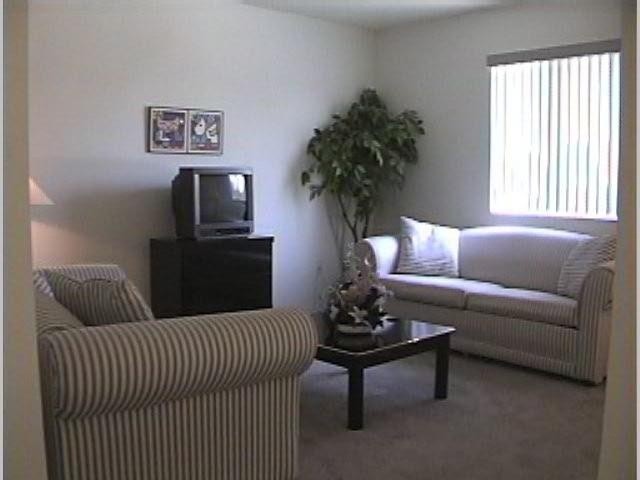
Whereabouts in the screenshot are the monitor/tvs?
[171,166,255,239]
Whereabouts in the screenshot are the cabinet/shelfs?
[148,234,275,317]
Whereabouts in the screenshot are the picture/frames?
[187,109,225,155]
[147,106,188,155]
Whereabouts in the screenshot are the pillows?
[394,215,460,277]
[558,232,616,297]
[42,268,155,327]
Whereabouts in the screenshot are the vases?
[332,337,385,353]
[332,323,396,336]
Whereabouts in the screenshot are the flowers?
[324,242,396,330]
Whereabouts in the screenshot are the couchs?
[361,223,618,385]
[34,262,319,480]
